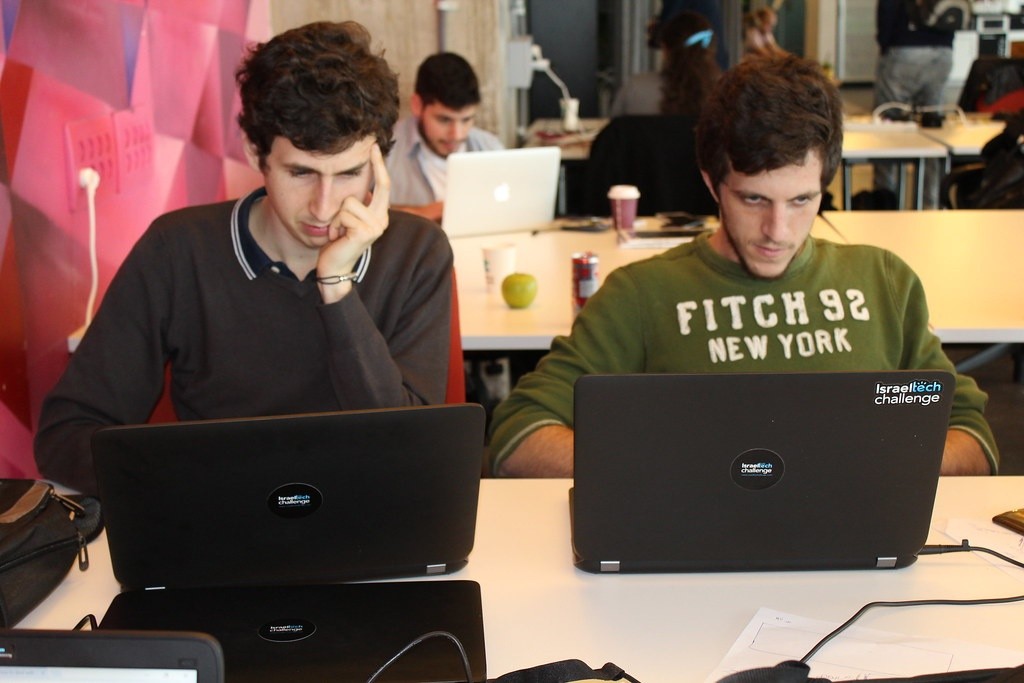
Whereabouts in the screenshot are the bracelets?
[315,273,357,284]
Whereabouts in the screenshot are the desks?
[522,110,948,216]
[451,214,851,415]
[821,207,1024,378]
[899,107,1024,210]
[6,474,1024,683]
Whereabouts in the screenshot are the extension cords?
[843,119,919,134]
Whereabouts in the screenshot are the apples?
[502,272,537,308]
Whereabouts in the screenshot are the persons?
[34,21,454,495]
[385,52,505,399]
[743,8,790,58]
[568,12,722,217]
[872,0,964,210]
[485,55,1000,478]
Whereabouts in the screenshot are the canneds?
[572,251,600,310]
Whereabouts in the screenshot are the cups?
[607,185,641,230]
[560,99,580,131]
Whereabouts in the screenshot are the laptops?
[0,405,489,683]
[440,146,561,237]
[568,370,955,574]
